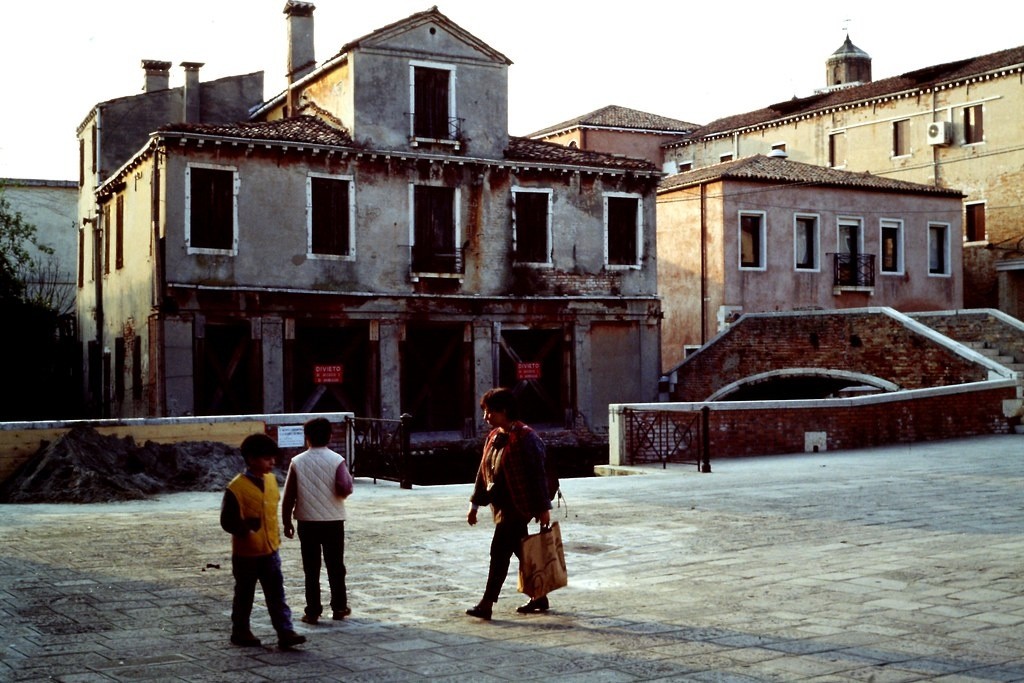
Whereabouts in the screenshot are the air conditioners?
[928,122,952,147]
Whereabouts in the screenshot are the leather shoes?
[465,605,492,620]
[516,596,549,613]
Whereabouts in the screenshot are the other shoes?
[301,615,317,625]
[334,606,351,620]
[230,630,260,647]
[278,631,306,649]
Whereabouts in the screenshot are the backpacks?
[485,427,560,502]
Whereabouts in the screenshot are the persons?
[465,388,553,620]
[282,417,354,624]
[220,434,306,649]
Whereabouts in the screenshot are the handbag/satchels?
[517,522,567,600]
